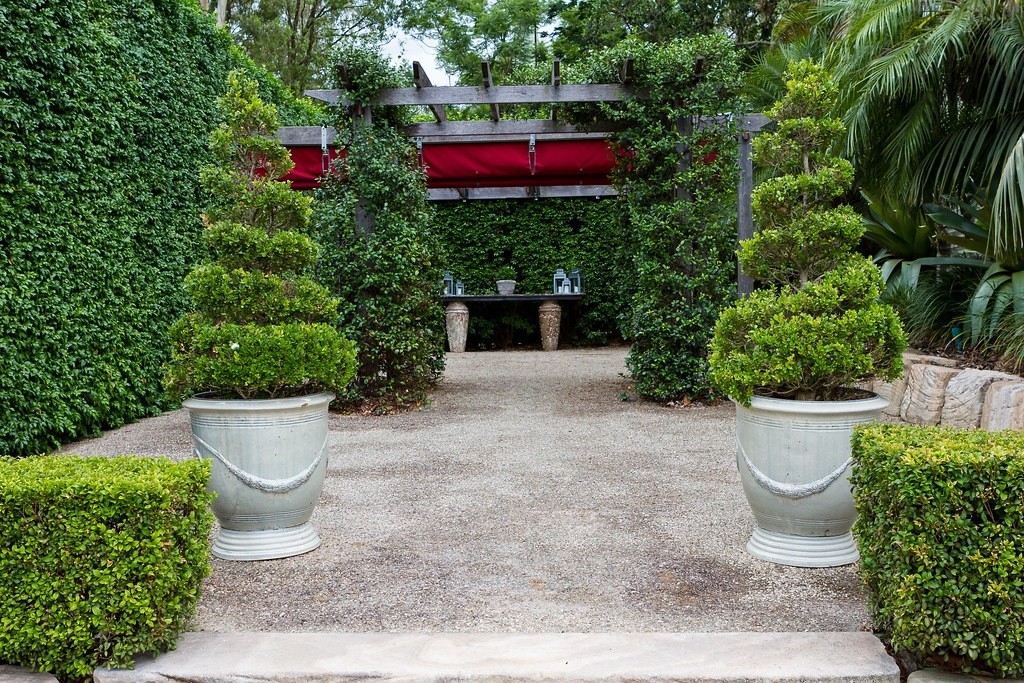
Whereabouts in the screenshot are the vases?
[538,301,562,352]
[445,301,469,353]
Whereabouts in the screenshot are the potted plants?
[169,66,358,562]
[493,266,517,296]
[707,57,909,569]
